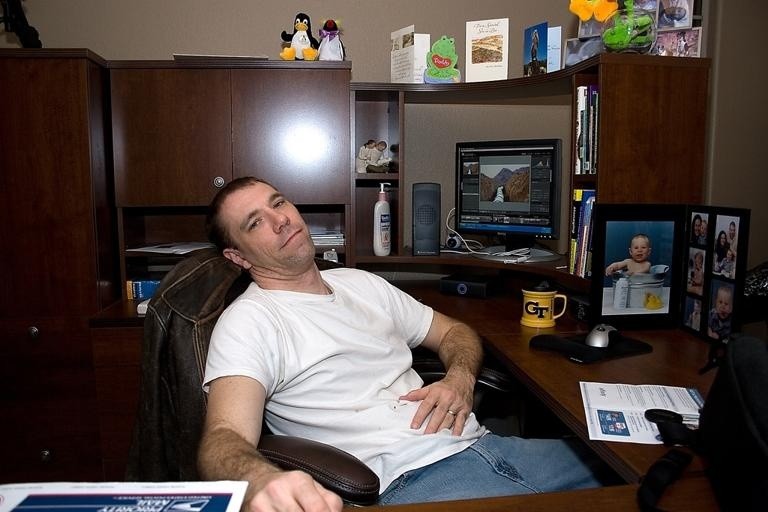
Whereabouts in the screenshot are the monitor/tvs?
[454,138,562,264]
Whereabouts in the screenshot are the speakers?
[412,182,441,256]
[440,273,494,297]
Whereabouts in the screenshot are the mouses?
[584,323,618,348]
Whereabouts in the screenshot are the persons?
[675,30,689,58]
[193,177,609,512]
[659,5,688,28]
[355,139,377,174]
[605,233,652,278]
[682,209,743,346]
[530,30,541,76]
[365,140,388,166]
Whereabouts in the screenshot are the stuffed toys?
[315,18,347,63]
[278,13,319,61]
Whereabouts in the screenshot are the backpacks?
[634,266,768,512]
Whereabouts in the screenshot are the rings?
[446,410,457,417]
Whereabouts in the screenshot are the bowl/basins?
[610,273,666,309]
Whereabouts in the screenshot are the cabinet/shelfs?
[85,308,145,482]
[101,53,354,301]
[355,53,712,295]
[0,48,120,483]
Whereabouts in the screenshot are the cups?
[519,287,567,330]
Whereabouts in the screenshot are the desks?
[334,271,732,510]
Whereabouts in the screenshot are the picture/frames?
[592,202,752,347]
[562,0,703,70]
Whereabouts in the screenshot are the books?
[574,86,600,175]
[307,229,345,247]
[567,188,596,279]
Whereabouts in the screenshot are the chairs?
[137,245,513,506]
